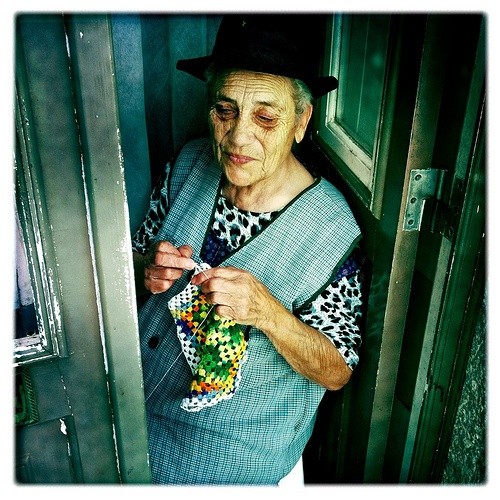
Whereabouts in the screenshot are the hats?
[174,15,342,101]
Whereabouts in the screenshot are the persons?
[130,15,366,485]
